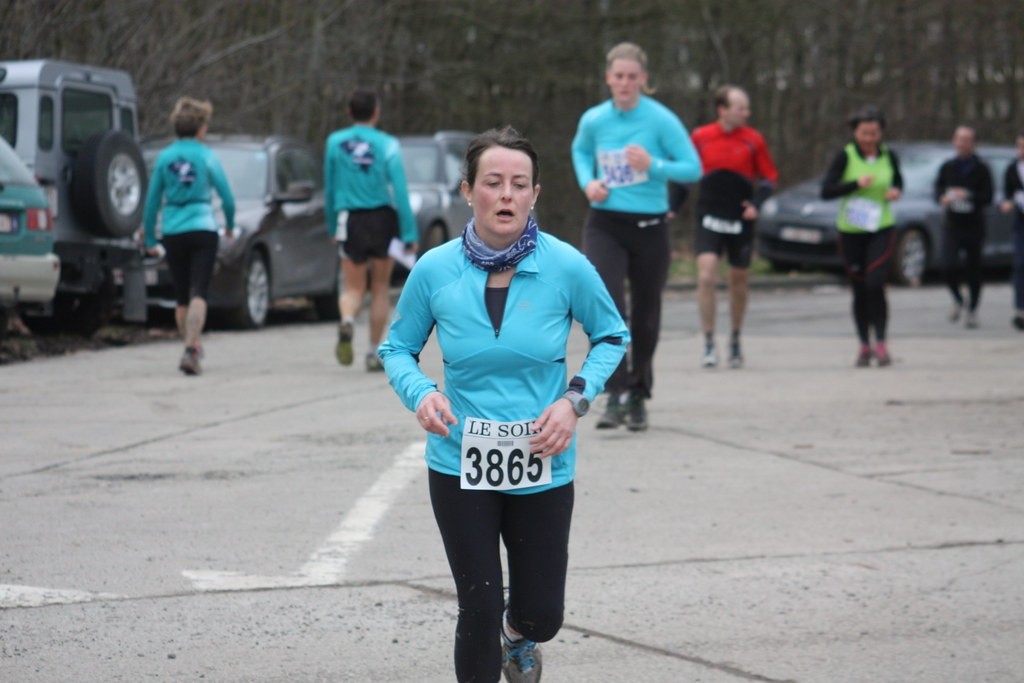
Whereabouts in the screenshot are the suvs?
[0,58,150,337]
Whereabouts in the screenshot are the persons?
[378,125,632,683]
[669,84,781,368]
[142,99,235,376]
[323,91,420,372]
[820,110,906,367]
[1001,133,1024,330]
[935,125,995,330]
[571,42,702,432]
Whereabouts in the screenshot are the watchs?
[563,391,590,417]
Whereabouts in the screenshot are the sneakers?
[627,395,649,431]
[499,587,542,683]
[181,343,204,375]
[597,396,624,428]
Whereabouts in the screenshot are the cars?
[366,128,480,290]
[753,140,1021,288]
[107,133,342,330]
[0,132,61,310]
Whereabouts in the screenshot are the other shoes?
[875,342,889,368]
[703,345,721,366]
[965,309,978,327]
[366,350,381,372]
[948,299,964,320]
[1014,317,1024,328]
[336,321,354,365]
[857,344,872,367]
[730,349,744,370]
